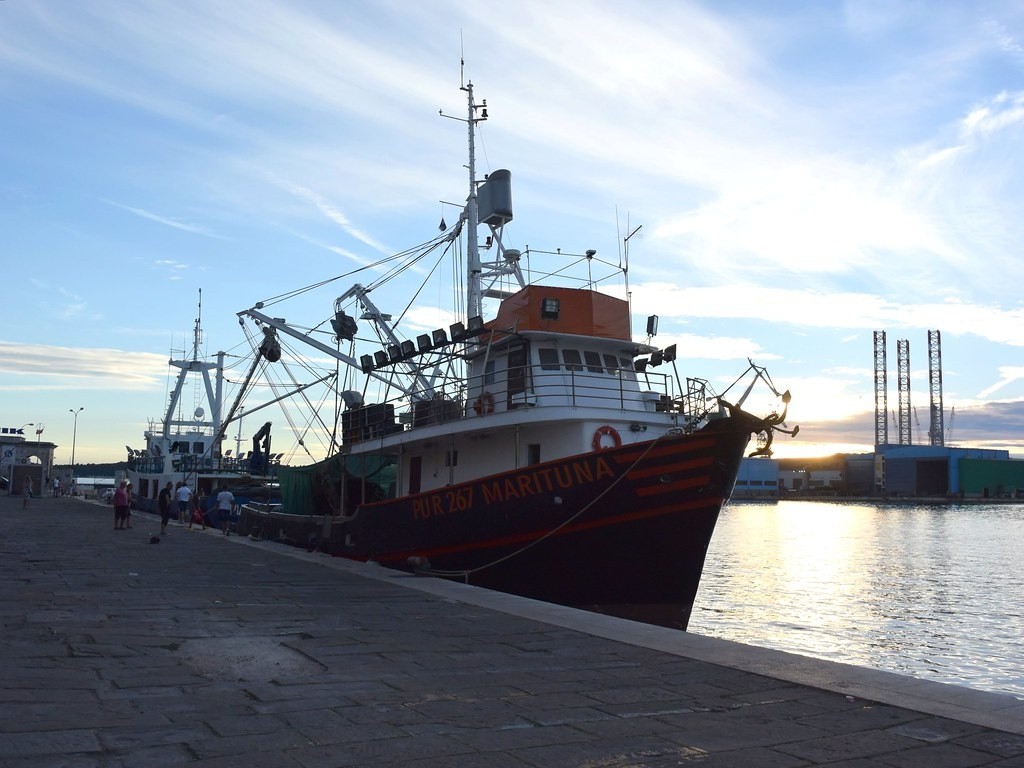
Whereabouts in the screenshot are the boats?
[239,57,799,630]
[114,288,282,531]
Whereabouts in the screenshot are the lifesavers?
[476,391,495,416]
[592,426,622,452]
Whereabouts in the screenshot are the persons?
[69,479,76,499]
[156,482,174,536]
[215,484,236,537]
[53,476,60,498]
[121,483,137,529]
[23,476,33,511]
[57,479,63,496]
[112,480,129,530]
[176,482,192,524]
